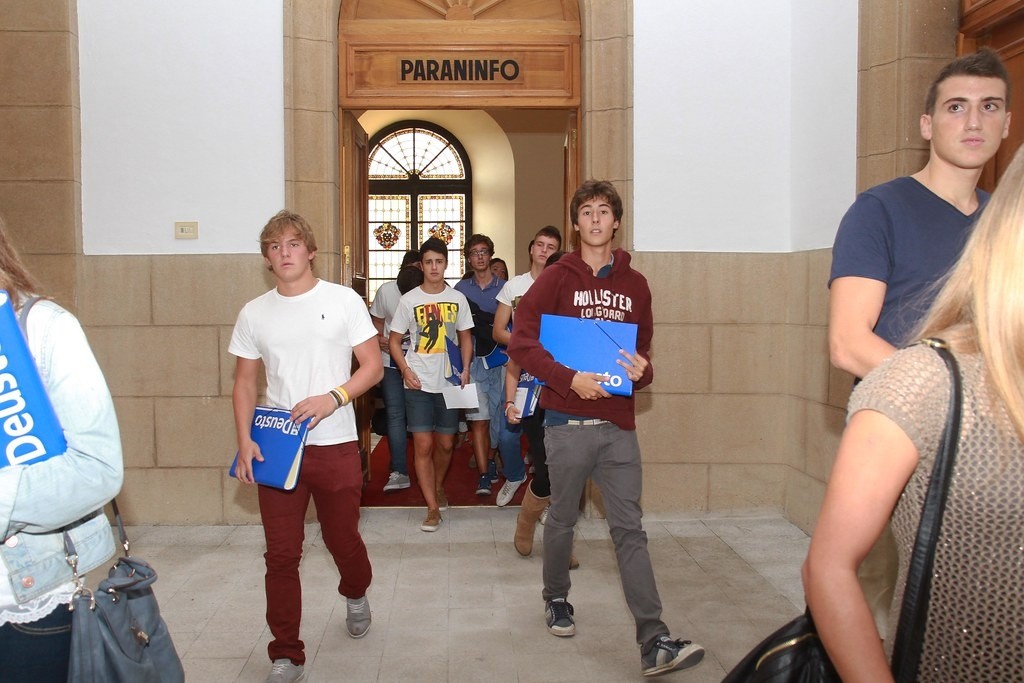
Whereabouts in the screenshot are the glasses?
[467,247,491,256]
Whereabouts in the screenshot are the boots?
[570,555,581,571]
[514,478,552,557]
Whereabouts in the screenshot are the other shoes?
[458,414,468,433]
[529,464,536,473]
[524,453,534,464]
[467,453,478,469]
[540,505,548,525]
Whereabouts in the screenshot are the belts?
[567,419,613,427]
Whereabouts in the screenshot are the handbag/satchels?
[723,614,843,683]
[57,557,186,683]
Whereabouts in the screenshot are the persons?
[0,217,124,683]
[828,49,1011,387]
[802,142,1024,683]
[228,209,384,683]
[507,180,704,676]
[369,226,579,570]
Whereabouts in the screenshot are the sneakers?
[383,470,412,495]
[476,474,493,497]
[262,659,306,683]
[420,508,442,532]
[640,633,707,678]
[344,596,374,639]
[543,594,576,637]
[488,458,500,484]
[435,486,449,511]
[495,474,527,507]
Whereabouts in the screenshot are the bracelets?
[328,386,349,408]
[505,404,514,416]
[401,366,411,377]
[506,401,514,404]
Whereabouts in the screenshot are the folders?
[482,322,513,370]
[514,369,541,419]
[0,290,68,467]
[539,313,639,396]
[230,406,312,490]
[444,335,463,386]
